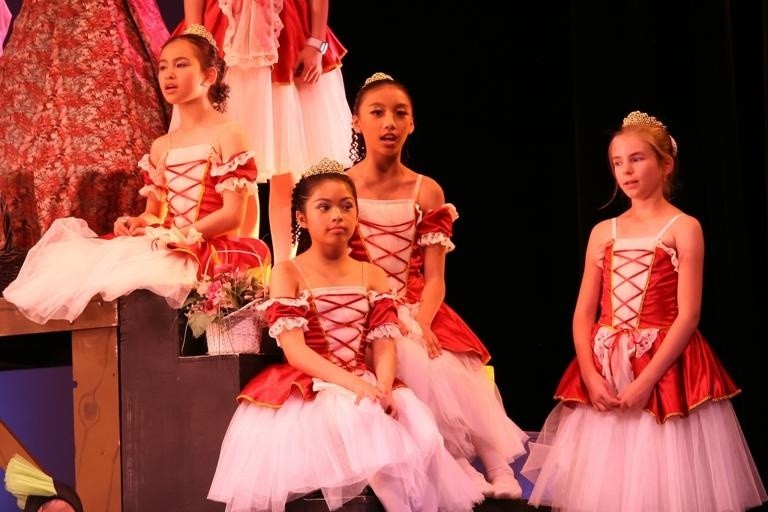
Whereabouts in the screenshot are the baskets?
[200,250,271,354]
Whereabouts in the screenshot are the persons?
[521,111,765,512]
[341,72,530,499]
[173,1,356,263]
[1,0,179,257]
[4,24,256,324]
[206,157,493,512]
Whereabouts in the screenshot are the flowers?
[181,262,270,357]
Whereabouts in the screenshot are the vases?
[205,298,263,354]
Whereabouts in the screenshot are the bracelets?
[305,37,329,56]
